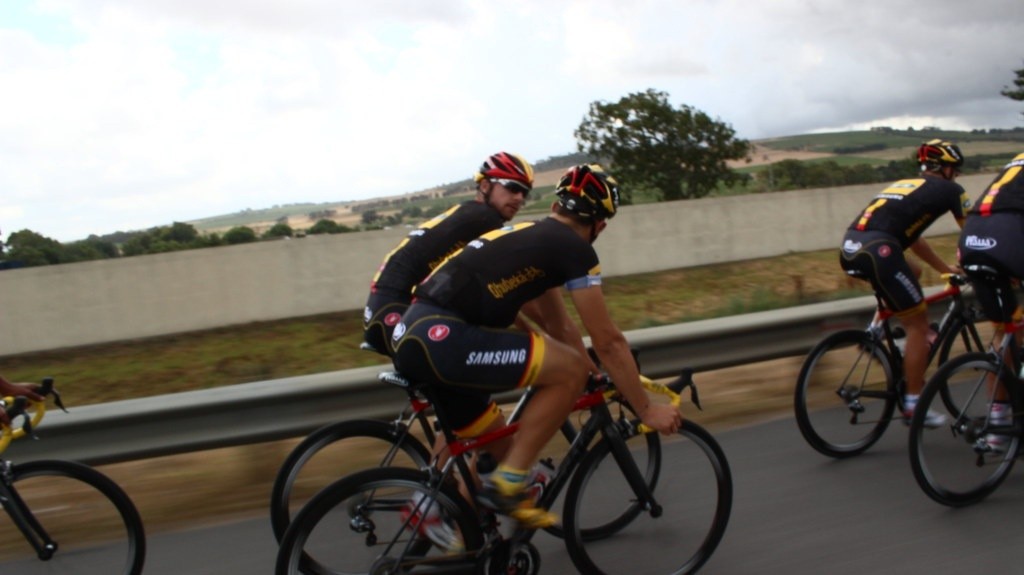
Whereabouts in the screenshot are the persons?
[956,152,1024,456]
[0,375,46,432]
[391,162,686,557]
[840,139,974,428]
[363,150,602,557]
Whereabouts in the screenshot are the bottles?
[476,452,498,487]
[927,323,939,350]
[892,327,906,357]
[533,457,555,498]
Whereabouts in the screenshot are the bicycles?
[793,273,1004,459]
[270,340,662,575]
[909,322,1023,509]
[274,366,734,575]
[0,375,147,575]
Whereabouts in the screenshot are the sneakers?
[400,490,456,552]
[444,529,465,558]
[987,419,1014,454]
[858,327,891,365]
[472,478,561,530]
[902,405,946,428]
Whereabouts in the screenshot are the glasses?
[489,178,531,199]
[947,165,962,177]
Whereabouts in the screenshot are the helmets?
[474,151,535,190]
[553,162,621,220]
[916,138,965,167]
